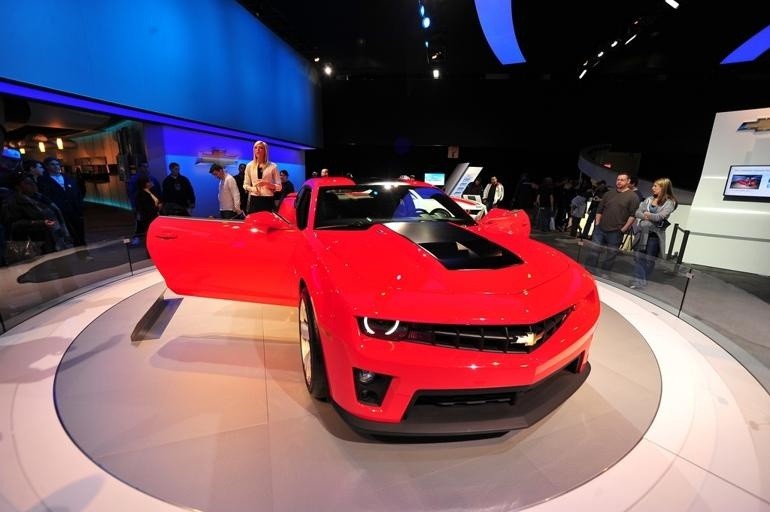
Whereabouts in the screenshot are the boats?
[194,145,243,168]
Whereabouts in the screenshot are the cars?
[359,176,488,221]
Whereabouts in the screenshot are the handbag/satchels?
[549,217,556,231]
[0,240,37,267]
[619,233,635,252]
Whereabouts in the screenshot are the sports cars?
[141,169,606,445]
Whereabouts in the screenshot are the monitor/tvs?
[721,165,770,202]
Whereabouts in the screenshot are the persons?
[320,168,329,177]
[1,138,296,266]
[468,168,679,288]
